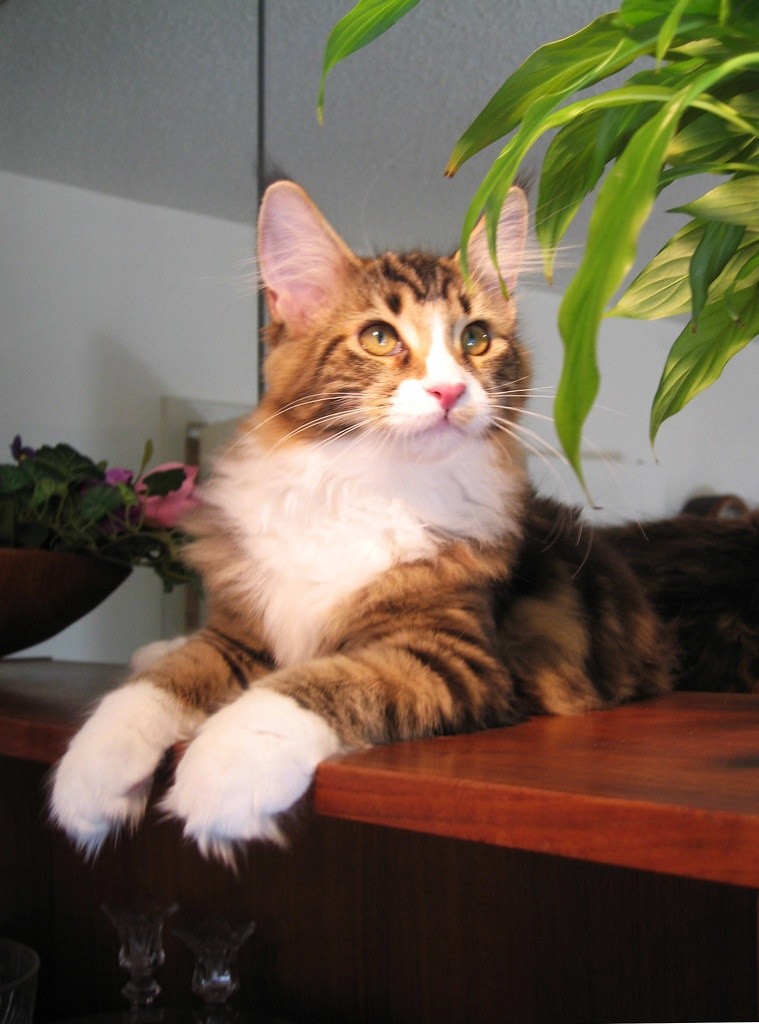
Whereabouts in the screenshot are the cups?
[100,901,181,1024]
[177,909,257,1024]
[0,938,41,1024]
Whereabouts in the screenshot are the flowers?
[0,434,206,600]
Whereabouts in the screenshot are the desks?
[0,660,759,1022]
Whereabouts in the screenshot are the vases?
[0,548,133,660]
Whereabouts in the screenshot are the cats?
[36,160,759,881]
